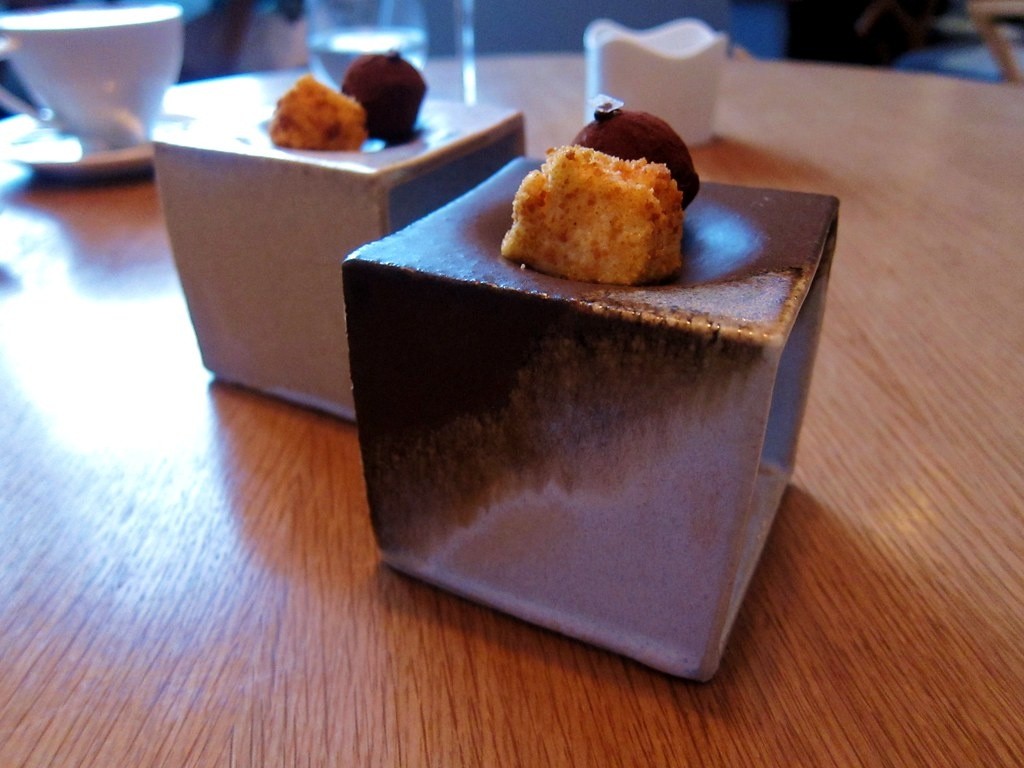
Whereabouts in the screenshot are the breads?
[499,144,685,286]
[267,73,367,150]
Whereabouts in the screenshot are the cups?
[585,19,728,145]
[0,7,184,145]
[375,0,478,109]
[305,0,430,94]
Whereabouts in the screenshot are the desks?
[0,53,1024,768]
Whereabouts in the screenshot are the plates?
[5,136,154,180]
